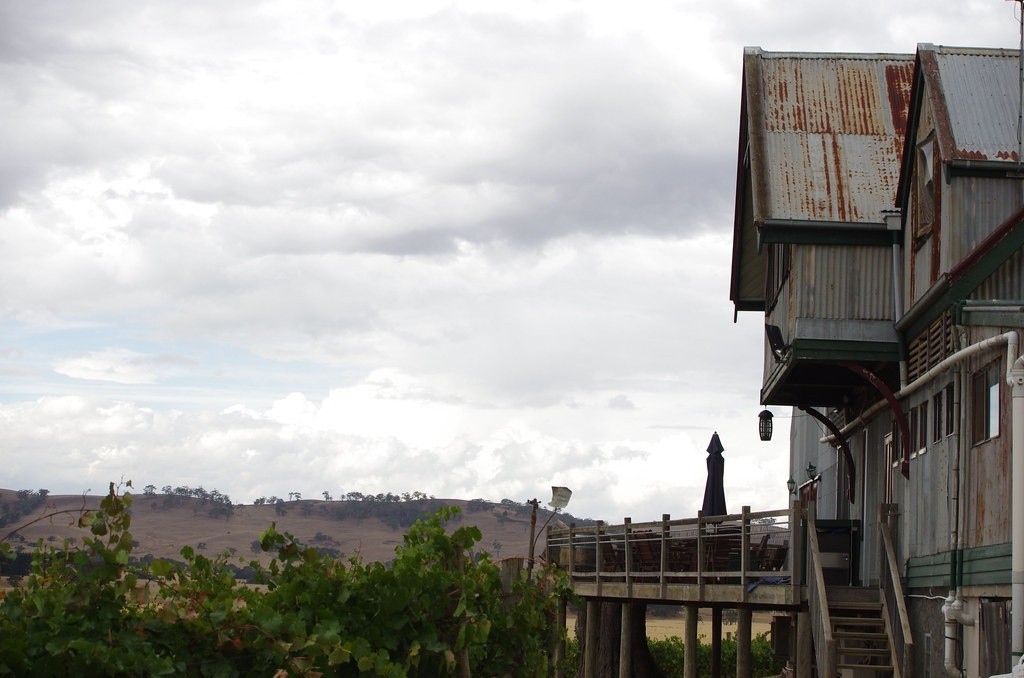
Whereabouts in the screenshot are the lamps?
[805,461,822,482]
[787,475,798,495]
[758,405,774,441]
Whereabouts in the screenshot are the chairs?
[601,523,788,584]
